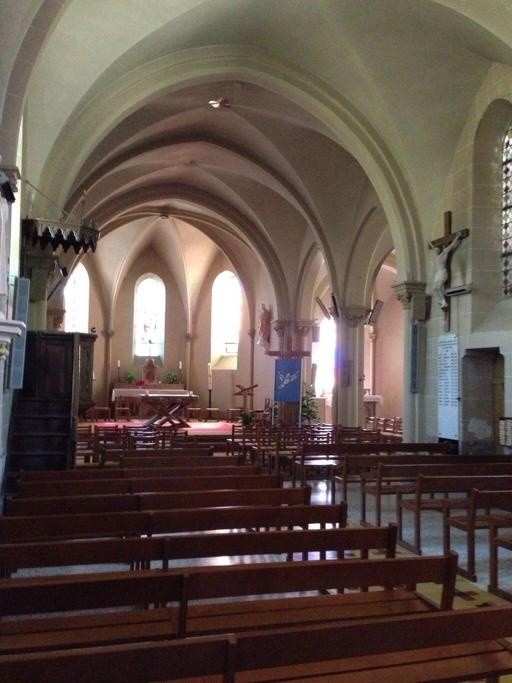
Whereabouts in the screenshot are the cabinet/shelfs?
[26,330,99,413]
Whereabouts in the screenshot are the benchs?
[1,383,512,683]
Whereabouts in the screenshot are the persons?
[258,301,270,342]
[426,231,461,308]
